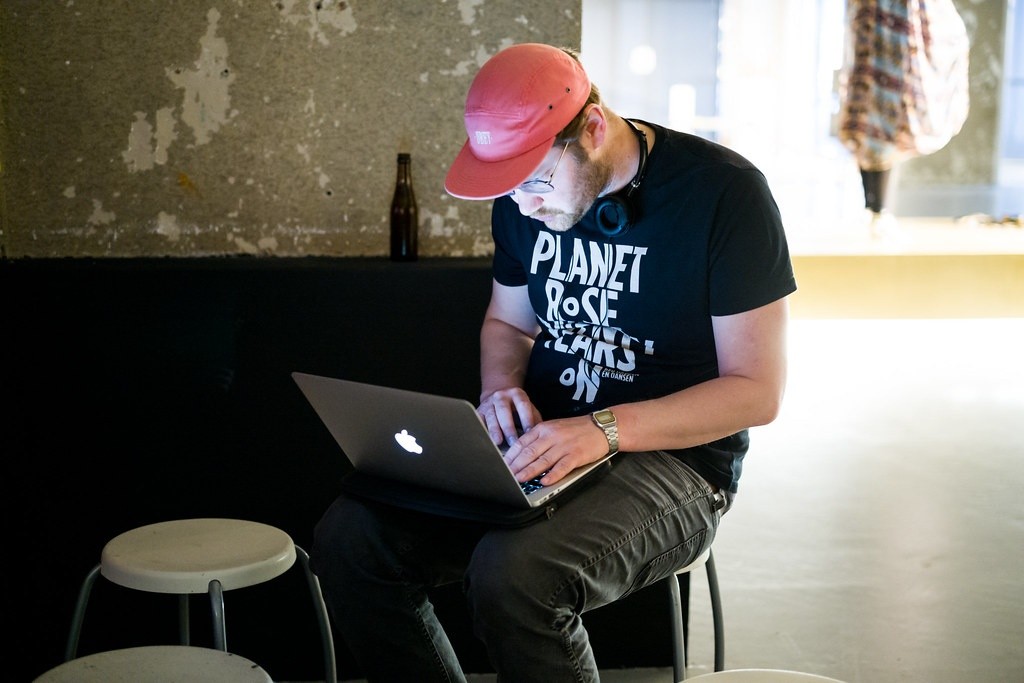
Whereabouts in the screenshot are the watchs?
[589,409,619,455]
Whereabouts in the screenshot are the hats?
[444,43,591,201]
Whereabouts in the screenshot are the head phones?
[596,116,649,236]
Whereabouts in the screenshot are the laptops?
[291,372,620,507]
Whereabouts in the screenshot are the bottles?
[389,153,418,259]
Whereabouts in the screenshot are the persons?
[311,42,797,683]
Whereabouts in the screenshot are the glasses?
[505,141,570,195]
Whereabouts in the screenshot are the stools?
[65,517,338,683]
[667,544,725,683]
[677,668,848,683]
[31,645,275,683]
